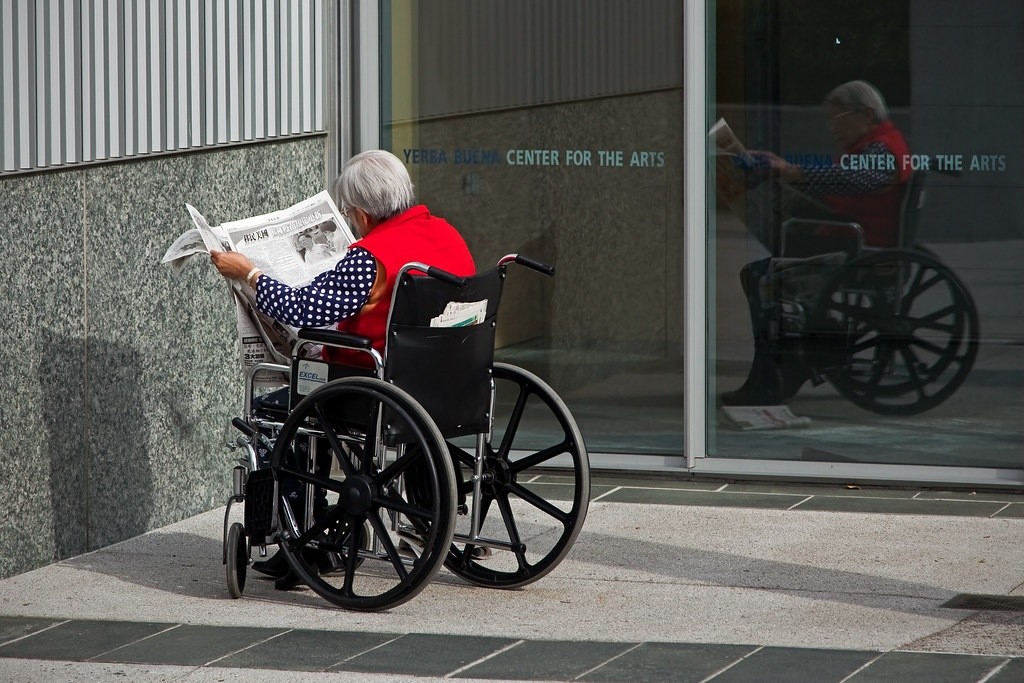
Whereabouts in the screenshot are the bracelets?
[247,268,262,285]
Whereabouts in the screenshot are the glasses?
[823,107,858,131]
[340,206,356,217]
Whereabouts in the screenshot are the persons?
[210,150,477,582]
[298,220,349,263]
[721,82,913,406]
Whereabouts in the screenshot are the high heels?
[745,358,828,406]
[253,542,339,590]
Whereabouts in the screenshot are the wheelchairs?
[221,253,590,615]
[758,159,979,418]
